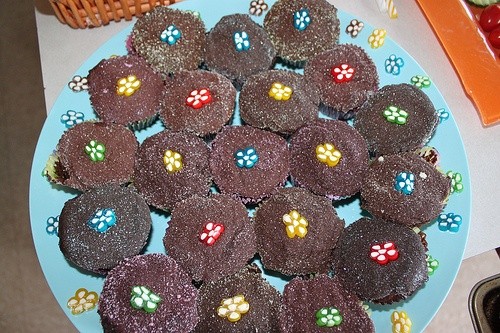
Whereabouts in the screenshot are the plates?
[27,0,476,333]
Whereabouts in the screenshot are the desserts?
[57,0,449,333]
